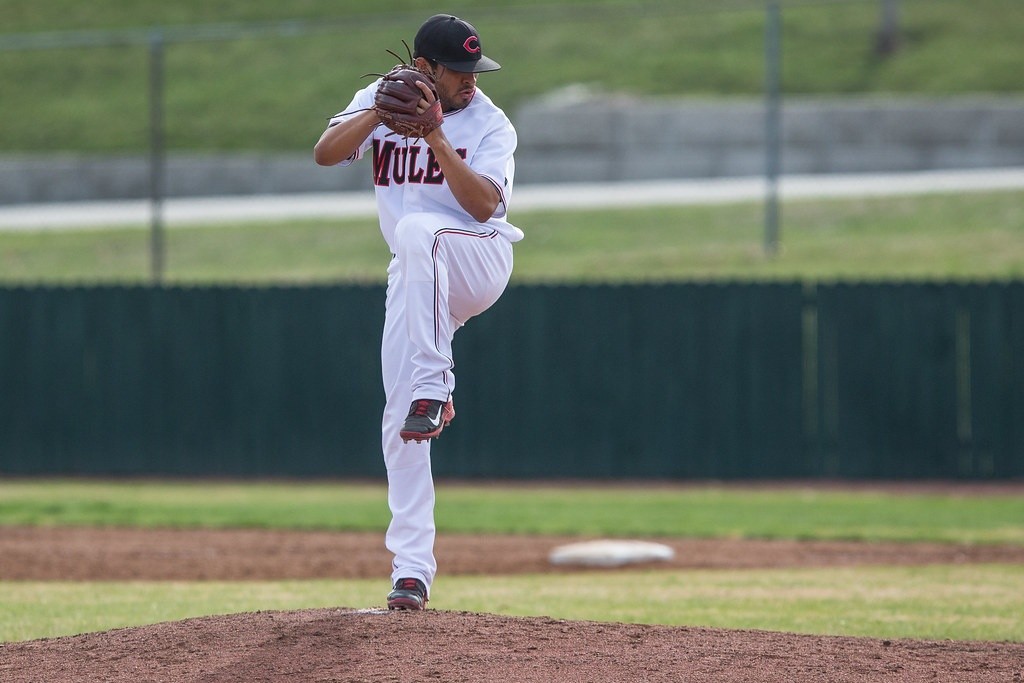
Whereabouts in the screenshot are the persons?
[314,14,524,612]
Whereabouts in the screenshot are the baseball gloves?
[374,63,444,139]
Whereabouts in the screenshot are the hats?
[413,14,502,73]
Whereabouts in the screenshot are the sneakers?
[400,399,455,444]
[387,578,429,610]
